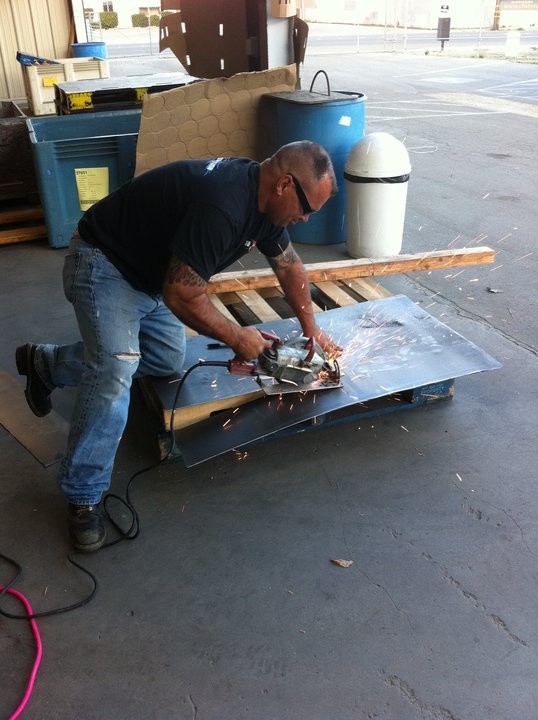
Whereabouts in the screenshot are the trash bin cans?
[343,131,413,259]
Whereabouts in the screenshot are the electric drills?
[226,329,332,389]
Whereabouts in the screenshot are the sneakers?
[64,496,107,553]
[15,341,55,418]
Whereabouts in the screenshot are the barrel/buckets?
[70,42,106,59]
[258,90,366,245]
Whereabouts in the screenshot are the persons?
[12,141,338,552]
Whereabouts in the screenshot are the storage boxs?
[22,56,110,116]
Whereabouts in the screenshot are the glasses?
[288,173,317,218]
